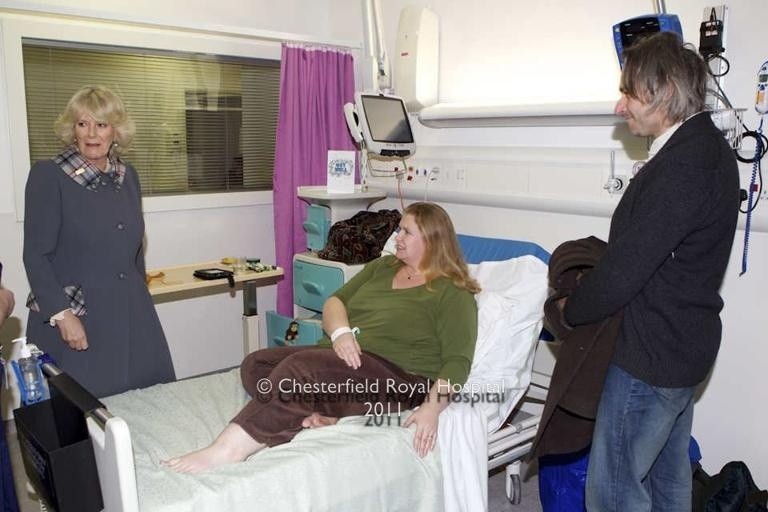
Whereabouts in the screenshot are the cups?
[231,257,247,274]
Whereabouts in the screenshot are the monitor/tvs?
[613,14,683,72]
[354,92,416,160]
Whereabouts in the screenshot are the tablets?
[195,268,233,278]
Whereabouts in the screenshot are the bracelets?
[329,326,356,343]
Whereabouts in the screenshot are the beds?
[35,227,579,512]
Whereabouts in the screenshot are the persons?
[158,202,483,475]
[1,261,16,327]
[547,30,741,511]
[27,83,176,399]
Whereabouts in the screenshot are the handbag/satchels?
[319,209,402,264]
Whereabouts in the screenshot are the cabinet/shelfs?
[266,184,388,366]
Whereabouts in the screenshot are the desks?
[141,257,284,367]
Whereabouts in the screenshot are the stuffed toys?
[284,321,299,341]
